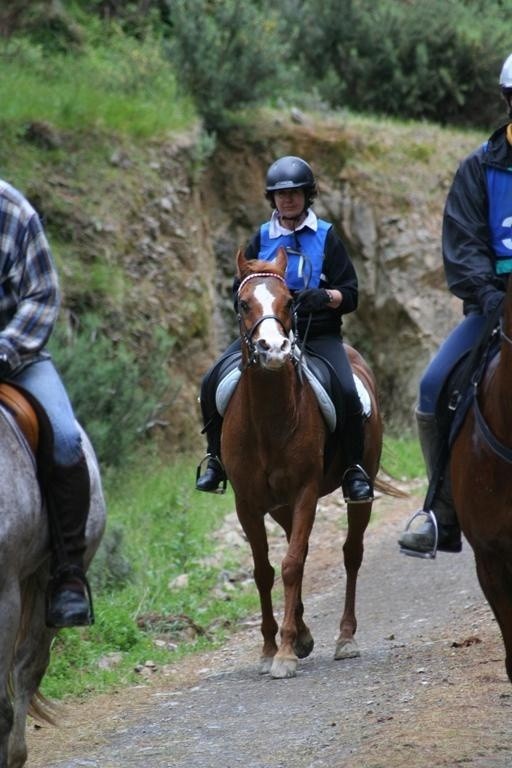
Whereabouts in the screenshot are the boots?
[45,496,93,628]
[339,406,375,506]
[398,408,462,553]
[196,425,220,491]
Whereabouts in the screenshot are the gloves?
[483,290,506,320]
[292,287,329,312]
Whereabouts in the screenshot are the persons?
[1,177,92,627]
[397,52,511,553]
[196,156,374,503]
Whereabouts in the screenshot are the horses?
[208,242,413,680]
[0,386,111,766]
[441,296,511,685]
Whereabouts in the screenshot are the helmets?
[266,156,315,189]
[499,52,512,88]
